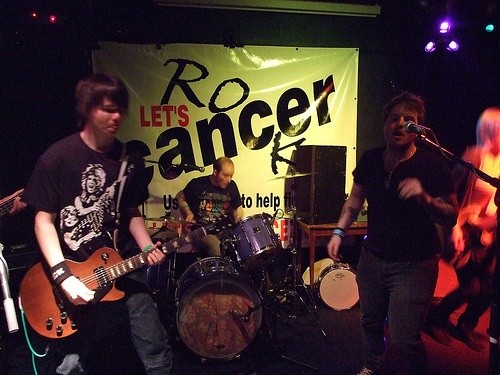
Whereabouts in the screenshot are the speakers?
[293,146,347,225]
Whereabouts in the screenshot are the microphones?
[184,164,206,172]
[269,211,278,226]
[231,308,249,323]
[404,121,431,133]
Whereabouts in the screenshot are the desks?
[294,220,369,290]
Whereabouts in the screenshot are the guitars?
[18,215,237,339]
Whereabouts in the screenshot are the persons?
[29,72,177,375]
[10,197,27,213]
[176,157,243,257]
[327,92,459,375]
[429,107,500,352]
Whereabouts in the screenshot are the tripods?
[246,177,330,375]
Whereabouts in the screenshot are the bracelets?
[332,228,346,239]
[50,261,73,285]
[182,206,189,209]
[144,245,153,252]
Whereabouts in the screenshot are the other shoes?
[421,320,453,345]
[356,366,376,375]
[454,316,485,352]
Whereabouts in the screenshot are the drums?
[220,214,282,273]
[301,257,360,312]
[176,258,263,361]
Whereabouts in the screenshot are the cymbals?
[144,216,183,223]
